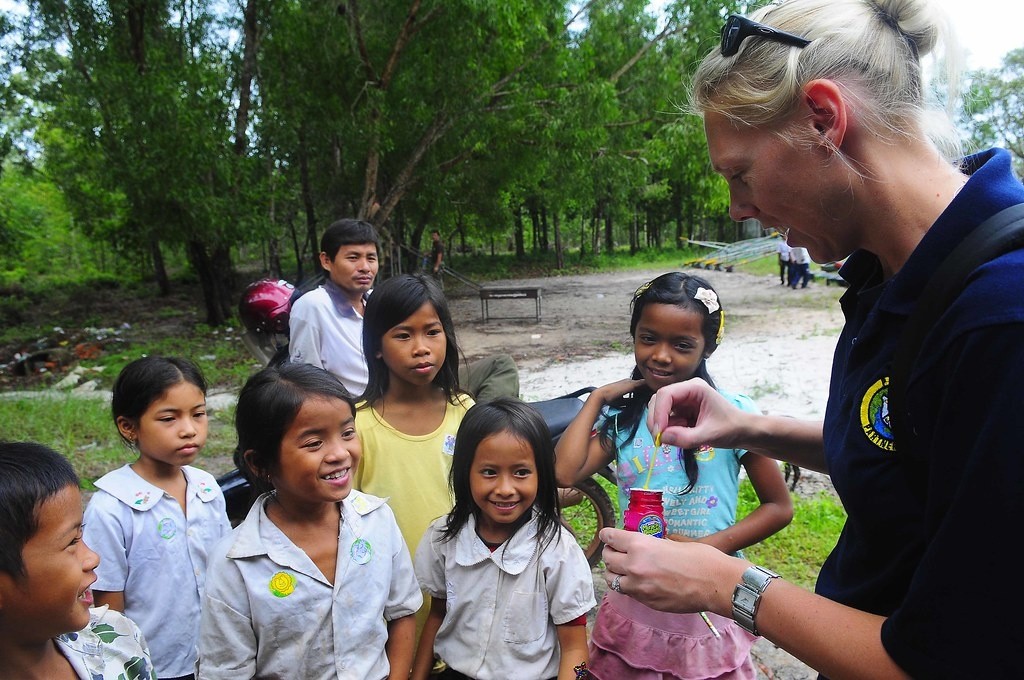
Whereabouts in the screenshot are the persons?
[414,398,599,679]
[424,229,444,289]
[347,275,478,654]
[556,271,794,680]
[192,363,423,680]
[81,357,233,679]
[777,234,791,285]
[288,220,520,406]
[0,442,157,680]
[791,242,810,288]
[601,0,1024,680]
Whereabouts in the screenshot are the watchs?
[731,566,783,636]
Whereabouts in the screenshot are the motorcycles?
[214,336,618,574]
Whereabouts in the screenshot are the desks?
[479,287,542,324]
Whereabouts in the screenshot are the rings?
[612,575,623,593]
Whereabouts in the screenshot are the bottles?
[624,488,667,540]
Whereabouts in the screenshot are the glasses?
[720,14,893,83]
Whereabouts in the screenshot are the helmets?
[239,278,296,331]
